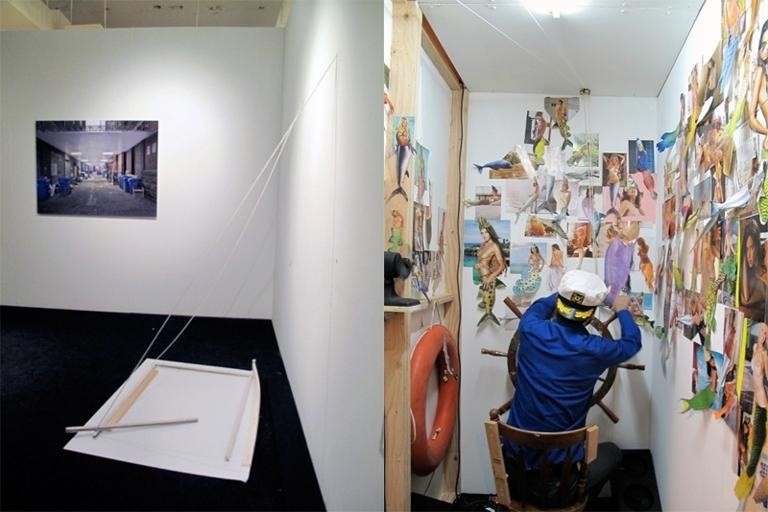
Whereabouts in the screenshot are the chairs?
[483,408,600,512]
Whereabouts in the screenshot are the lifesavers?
[409,324,460,471]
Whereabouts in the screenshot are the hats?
[557,269,608,322]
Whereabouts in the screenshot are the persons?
[383,0,767,512]
